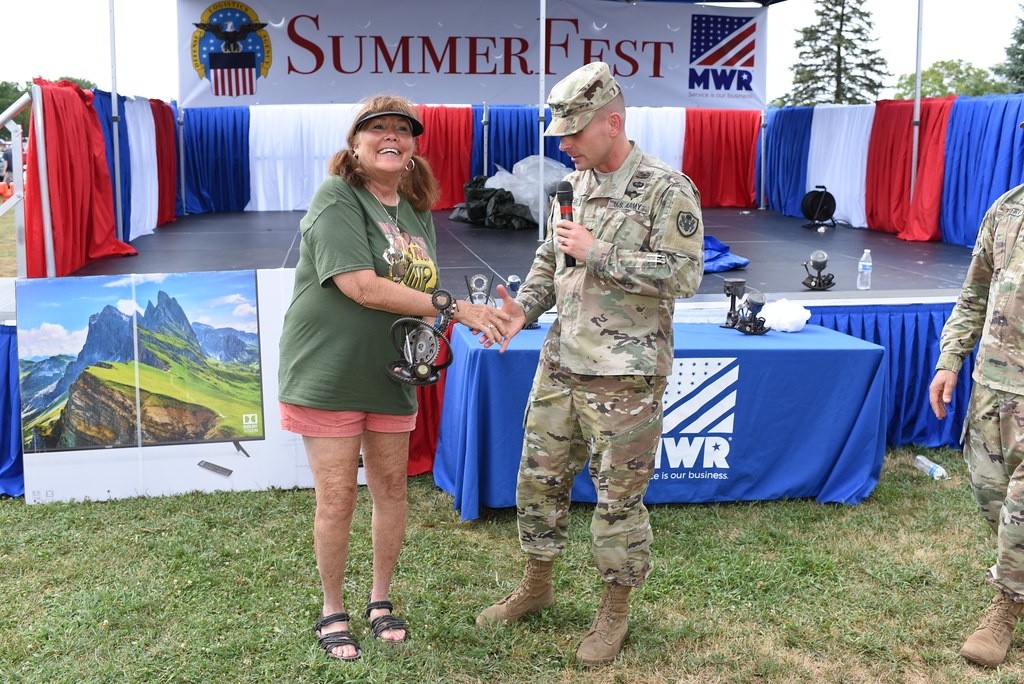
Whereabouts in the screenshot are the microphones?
[556,181,577,267]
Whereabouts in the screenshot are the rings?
[487,324,493,328]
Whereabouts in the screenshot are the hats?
[544,61,621,136]
[353,108,424,137]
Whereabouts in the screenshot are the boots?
[960,590,1024,666]
[575,581,631,663]
[477,558,554,628]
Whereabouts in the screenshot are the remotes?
[198,460,232,477]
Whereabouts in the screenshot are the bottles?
[913,454,951,482]
[857,248,872,290]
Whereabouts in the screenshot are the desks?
[432,323,886,522]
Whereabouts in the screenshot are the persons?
[473,60,705,665]
[278,95,513,661]
[929,121,1023,667]
[2,149,13,183]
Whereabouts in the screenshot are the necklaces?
[370,188,399,232]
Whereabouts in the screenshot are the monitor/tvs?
[15,267,265,454]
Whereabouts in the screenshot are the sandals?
[361,595,407,644]
[313,613,361,661]
